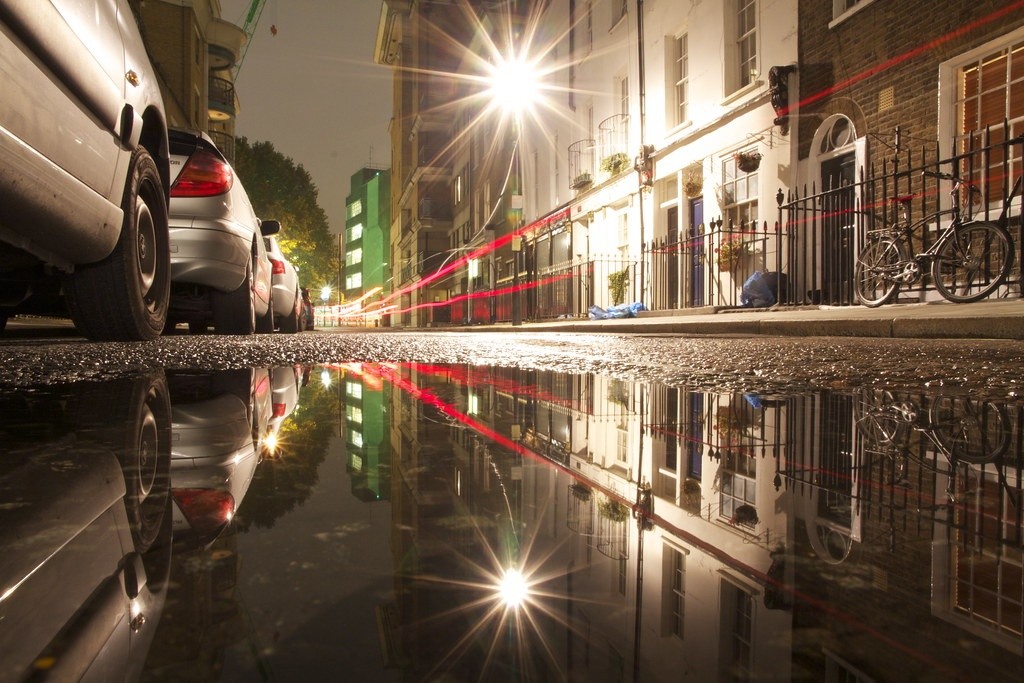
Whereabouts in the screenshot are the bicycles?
[855,170,1016,309]
[852,390,1014,504]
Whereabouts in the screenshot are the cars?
[262,235,302,334]
[0,0,176,342]
[0,368,317,683]
[166,125,282,335]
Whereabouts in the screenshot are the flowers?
[688,171,702,184]
[715,234,742,258]
[733,151,762,160]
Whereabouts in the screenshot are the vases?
[719,255,736,272]
[737,161,760,173]
[685,182,703,197]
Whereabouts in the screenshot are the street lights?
[320,286,332,327]
[483,55,546,326]
